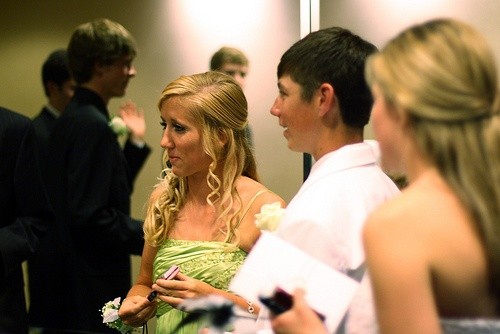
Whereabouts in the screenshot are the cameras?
[150,266,181,302]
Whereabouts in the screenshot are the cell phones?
[259,286,326,324]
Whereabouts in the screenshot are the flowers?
[108,114,131,151]
[256,202,288,229]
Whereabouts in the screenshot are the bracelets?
[245,299,255,315]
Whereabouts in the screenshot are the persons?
[0,105,33,334]
[99,72,285,334]
[207,47,254,149]
[148,27,404,334]
[32,18,155,334]
[227,17,500,334]
[25,48,79,334]
